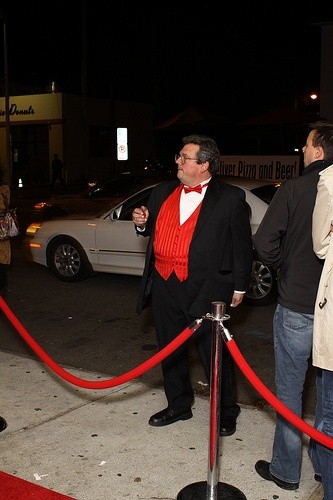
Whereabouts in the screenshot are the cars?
[23,175,281,307]
[31,174,156,221]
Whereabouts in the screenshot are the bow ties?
[182,182,208,194]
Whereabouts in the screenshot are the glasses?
[175,154,199,165]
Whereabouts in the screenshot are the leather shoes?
[148,406,193,426]
[219,405,241,436]
[255,460,299,490]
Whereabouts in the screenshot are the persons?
[253,121,333,490]
[0,160,11,298]
[132,135,252,436]
[312,165,333,500]
[47,153,70,194]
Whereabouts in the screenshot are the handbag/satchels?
[0,208,23,240]
